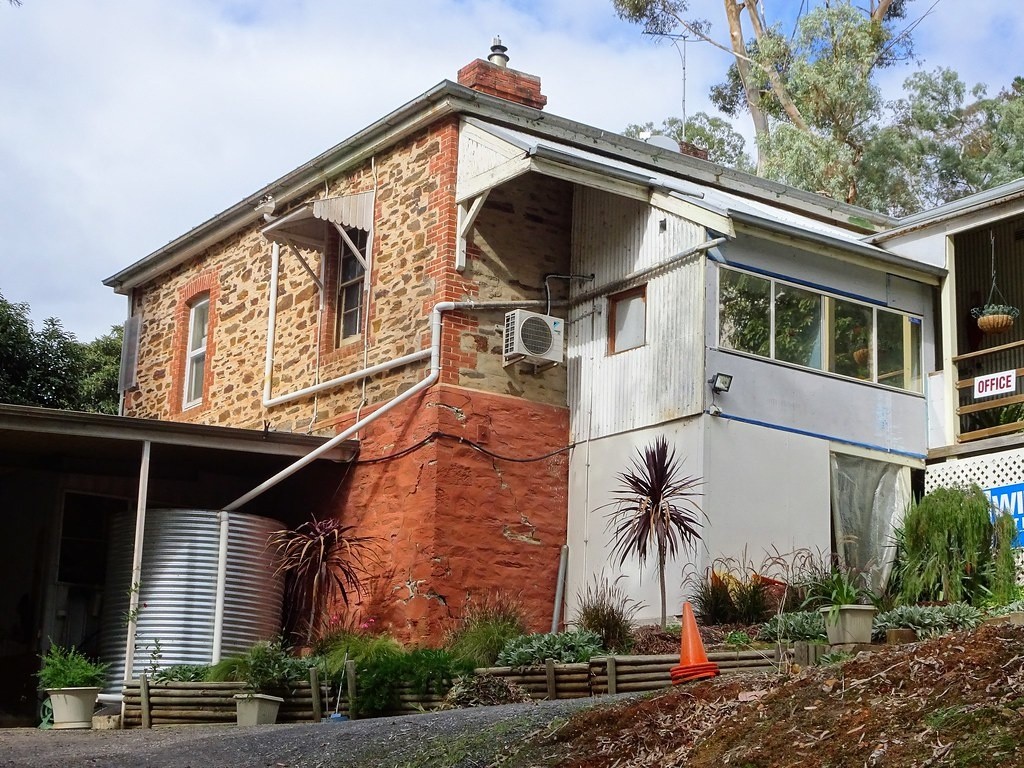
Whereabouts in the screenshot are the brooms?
[331,653,349,722]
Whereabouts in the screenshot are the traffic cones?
[671,602,721,688]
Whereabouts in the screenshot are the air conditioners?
[502,308,564,366]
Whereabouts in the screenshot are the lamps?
[708,371,734,394]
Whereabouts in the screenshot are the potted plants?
[232,633,296,730]
[800,563,885,646]
[31,634,113,731]
[972,303,1021,335]
